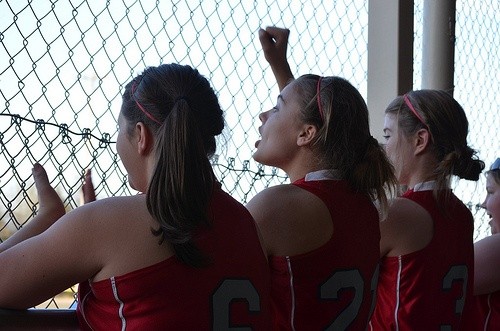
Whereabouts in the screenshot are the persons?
[0,63,270,331]
[473,158,500,331]
[367,90,485,331]
[246,27,400,331]
